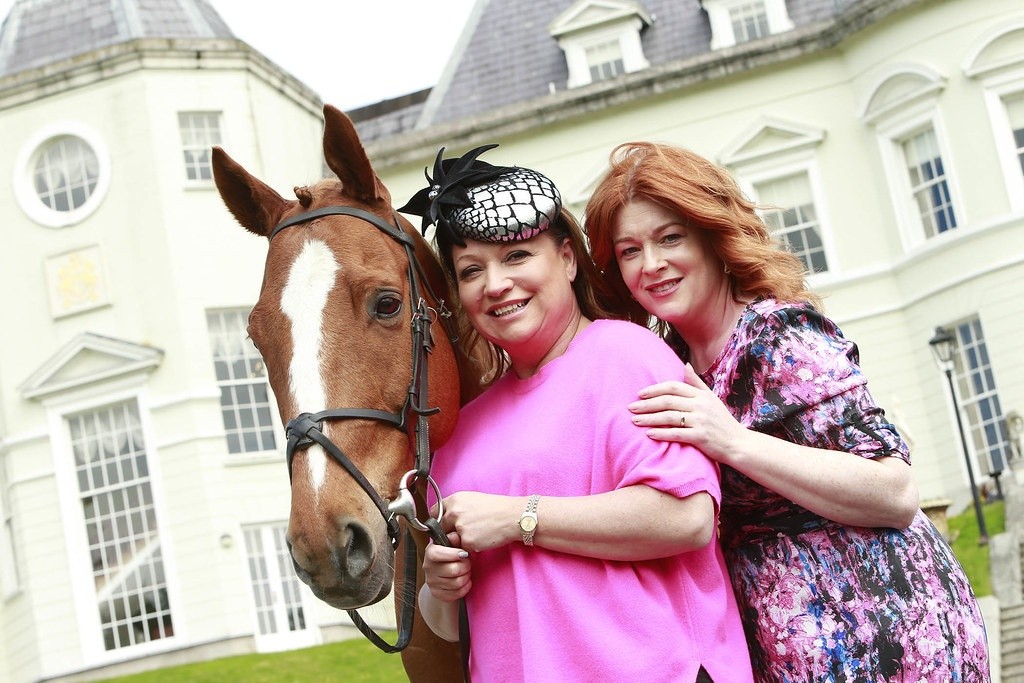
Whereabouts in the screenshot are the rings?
[678,411,686,428]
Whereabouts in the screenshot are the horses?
[209,104,469,682]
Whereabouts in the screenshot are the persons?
[395,145,754,683]
[583,140,993,683]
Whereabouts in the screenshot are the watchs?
[517,493,541,546]
